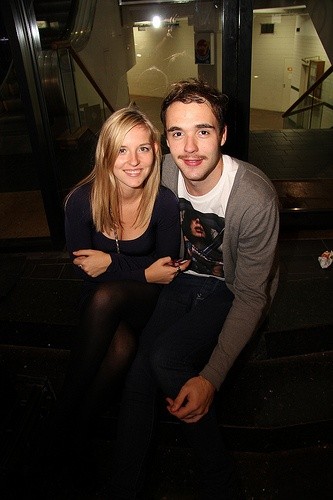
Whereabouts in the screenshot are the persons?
[106,78,283,500]
[42,107,191,427]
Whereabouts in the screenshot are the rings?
[176,266,182,274]
